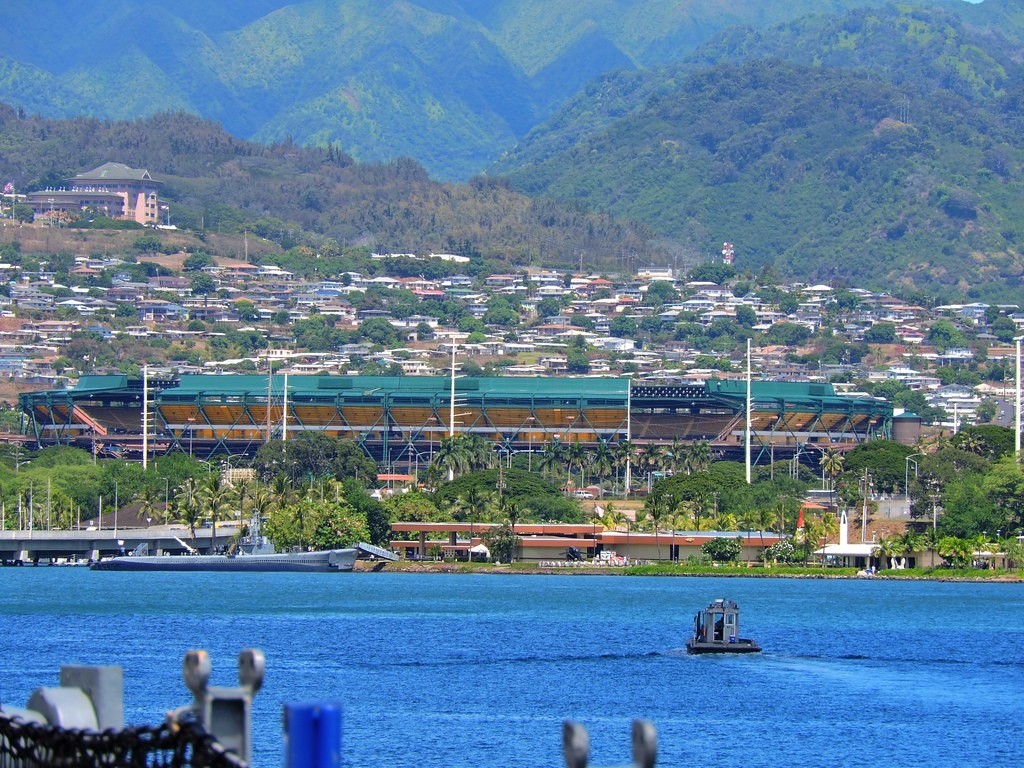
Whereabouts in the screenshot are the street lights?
[16,460,31,473]
[804,442,825,490]
[905,453,928,501]
[906,457,918,479]
[414,451,436,489]
[982,530,987,539]
[793,451,814,481]
[228,453,250,480]
[996,529,1001,540]
[200,459,212,479]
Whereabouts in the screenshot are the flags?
[594,504,605,518]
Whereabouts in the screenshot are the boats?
[90,535,360,571]
[684,597,763,655]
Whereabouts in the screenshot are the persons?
[28,520,31,530]
[972,557,996,571]
[118,541,253,560]
[871,565,876,575]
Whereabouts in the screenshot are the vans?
[573,491,594,500]
